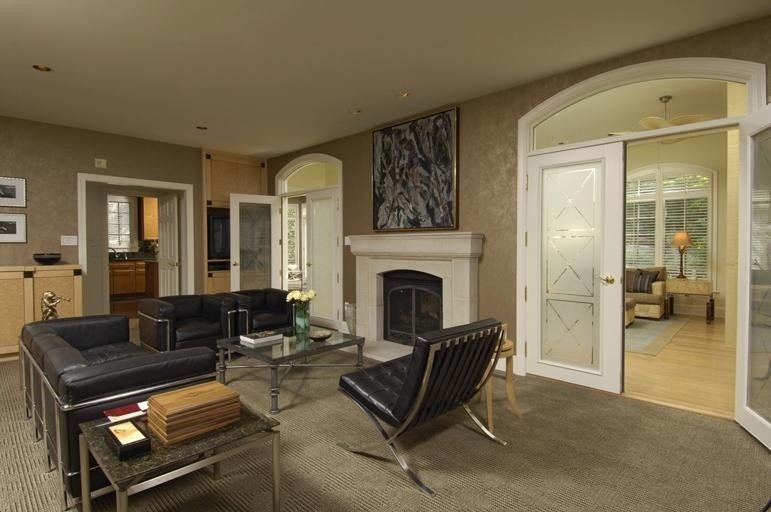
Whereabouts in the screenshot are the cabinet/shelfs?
[194,270,230,294]
[182,148,267,207]
[0,265,84,356]
[109,259,158,295]
[142,197,160,240]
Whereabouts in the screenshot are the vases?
[292,303,311,333]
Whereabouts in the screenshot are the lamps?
[671,232,694,278]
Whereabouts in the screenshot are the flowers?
[285,289,318,327]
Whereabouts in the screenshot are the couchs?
[218,287,298,345]
[625,266,666,321]
[18,312,221,512]
[135,292,235,362]
[624,297,637,327]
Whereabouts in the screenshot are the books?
[241,329,284,344]
[241,338,284,349]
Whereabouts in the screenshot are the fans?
[607,94,721,147]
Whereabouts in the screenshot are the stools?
[481,338,522,432]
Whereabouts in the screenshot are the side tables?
[665,291,720,325]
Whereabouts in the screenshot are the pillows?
[634,268,660,293]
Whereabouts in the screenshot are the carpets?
[624,313,695,356]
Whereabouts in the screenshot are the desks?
[76,391,284,511]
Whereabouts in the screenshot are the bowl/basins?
[32,252,62,265]
[308,330,333,343]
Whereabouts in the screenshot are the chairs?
[335,316,511,497]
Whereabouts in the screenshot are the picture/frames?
[370,106,460,232]
[0,176,27,208]
[0,213,28,244]
